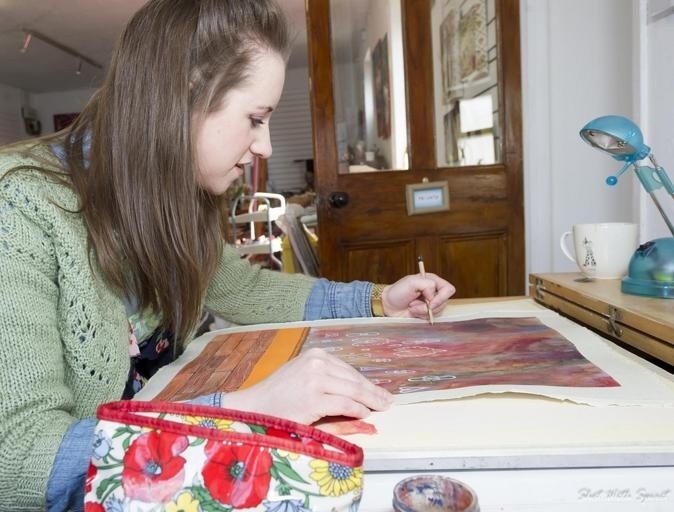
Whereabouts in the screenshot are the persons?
[0,0,456,512]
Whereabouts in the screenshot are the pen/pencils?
[417,255,434,325]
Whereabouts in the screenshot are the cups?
[560,222,638,279]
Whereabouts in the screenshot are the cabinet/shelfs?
[226,192,286,275]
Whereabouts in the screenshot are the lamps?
[581,111,674,299]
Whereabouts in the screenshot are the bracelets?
[371,283,386,317]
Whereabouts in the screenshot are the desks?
[226,292,673,510]
